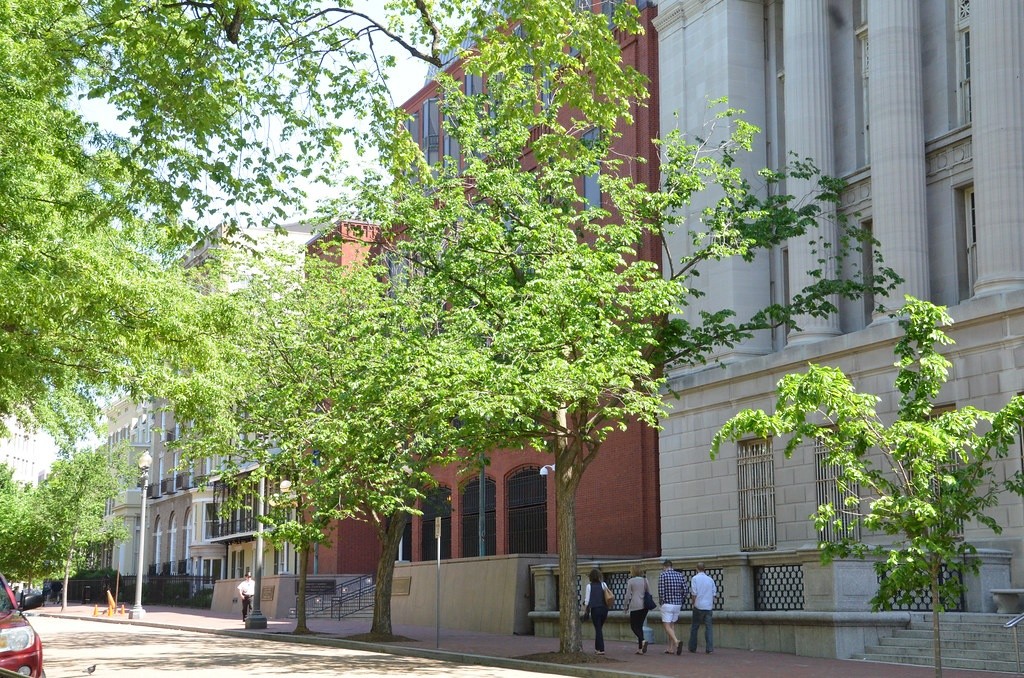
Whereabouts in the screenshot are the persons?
[687,562,717,655]
[624,565,655,655]
[237,572,256,622]
[42,577,52,608]
[581,568,616,655]
[658,559,688,656]
[50,576,64,604]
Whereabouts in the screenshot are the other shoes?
[641,640,648,653]
[688,649,696,654]
[594,649,600,654]
[677,641,683,655]
[705,650,714,655]
[664,649,674,654]
[596,651,605,655]
[634,650,643,655]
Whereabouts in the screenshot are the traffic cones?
[121,605,126,616]
[107,606,114,617]
[93,604,99,617]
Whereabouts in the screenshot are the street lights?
[130,450,153,619]
[280,479,292,571]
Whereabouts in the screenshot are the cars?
[0,574,46,678]
[21,588,43,610]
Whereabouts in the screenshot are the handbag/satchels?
[579,613,589,624]
[602,582,614,608]
[644,591,657,610]
